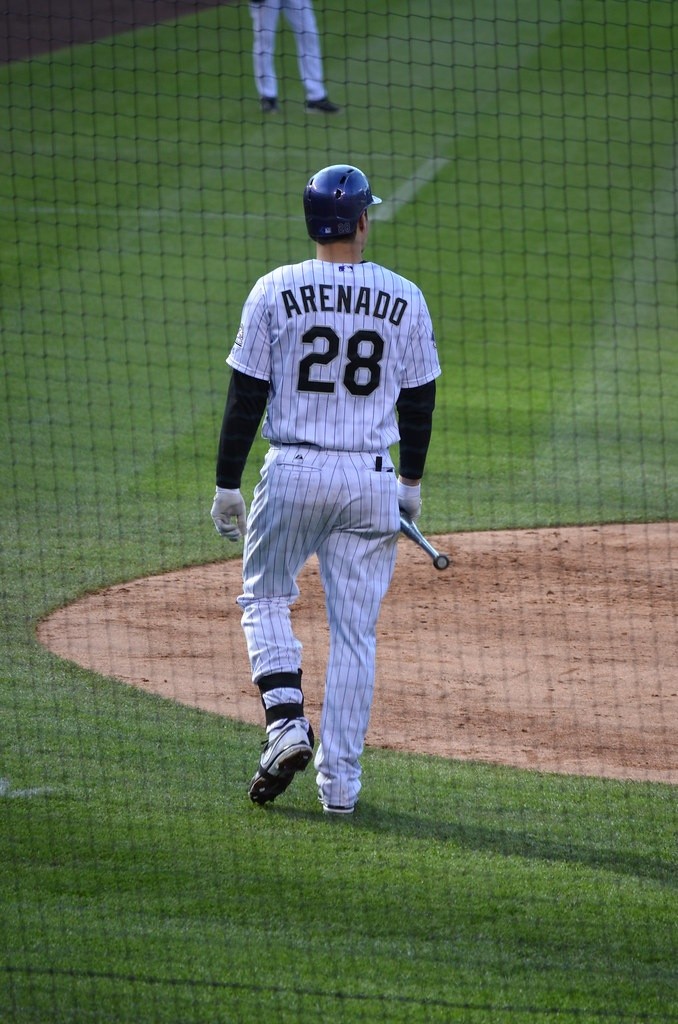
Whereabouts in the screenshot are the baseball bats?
[399,508,451,572]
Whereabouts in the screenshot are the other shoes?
[304,97,343,115]
[260,96,277,113]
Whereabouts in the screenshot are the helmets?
[303,164,383,238]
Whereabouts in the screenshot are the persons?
[210,164,441,812]
[248,0,339,113]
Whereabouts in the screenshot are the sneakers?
[249,720,314,806]
[317,790,355,816]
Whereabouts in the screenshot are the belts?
[281,441,321,447]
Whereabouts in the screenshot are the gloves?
[210,484,248,542]
[396,479,421,521]
